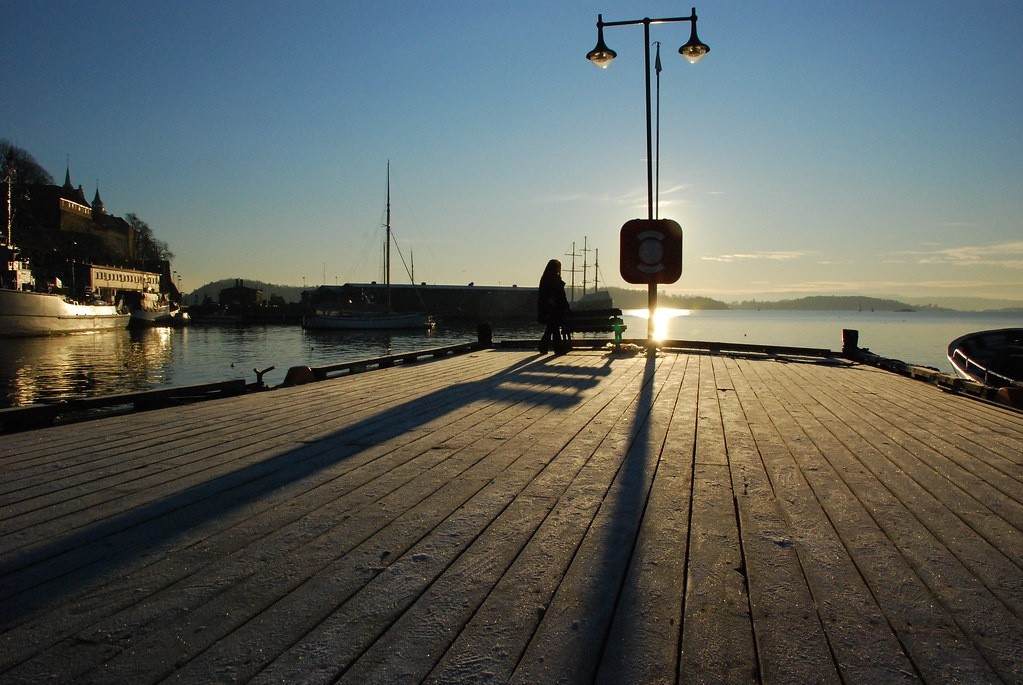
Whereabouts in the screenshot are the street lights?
[585,5,712,349]
[65,258,76,295]
[172,271,182,291]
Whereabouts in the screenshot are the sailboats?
[302,157,437,331]
[133,258,180,321]
[0,163,131,334]
[561,235,612,310]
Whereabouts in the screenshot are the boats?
[946,328,1022,398]
[170,308,191,323]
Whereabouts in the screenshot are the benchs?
[543,307,627,349]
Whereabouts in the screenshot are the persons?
[538,259,572,356]
